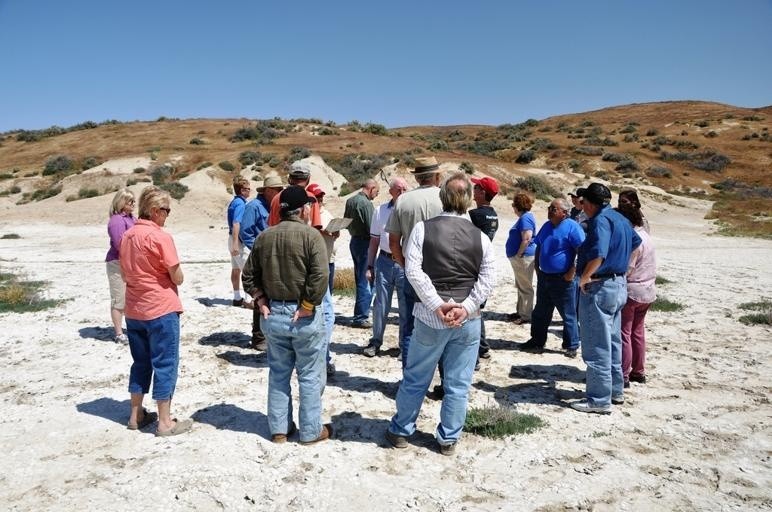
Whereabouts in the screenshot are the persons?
[568,187,591,235]
[519,198,581,359]
[468,177,499,371]
[505,192,537,326]
[223,161,333,352]
[241,184,334,445]
[611,190,657,389]
[569,183,642,413]
[344,156,445,362]
[118,185,194,436]
[383,174,498,456]
[104,187,136,345]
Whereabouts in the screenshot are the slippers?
[128,410,157,429]
[156,418,192,436]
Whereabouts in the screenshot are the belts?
[591,273,623,277]
[380,249,397,261]
[271,299,297,302]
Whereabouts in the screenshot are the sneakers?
[327,364,335,376]
[255,343,267,350]
[629,375,645,383]
[352,321,373,328]
[565,348,576,357]
[612,396,624,402]
[364,344,380,356]
[273,422,296,442]
[571,401,611,413]
[474,362,480,370]
[479,351,490,358]
[115,334,129,344]
[519,339,543,349]
[434,385,445,394]
[303,424,333,443]
[242,300,254,309]
[385,427,408,447]
[504,313,530,324]
[436,438,456,456]
[233,298,245,306]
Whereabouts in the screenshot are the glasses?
[129,200,135,204]
[619,199,628,203]
[548,207,562,213]
[160,208,170,213]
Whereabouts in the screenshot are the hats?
[280,185,316,211]
[290,161,311,176]
[577,183,611,205]
[409,156,445,175]
[256,176,287,192]
[306,184,325,197]
[471,177,499,197]
[568,188,579,197]
